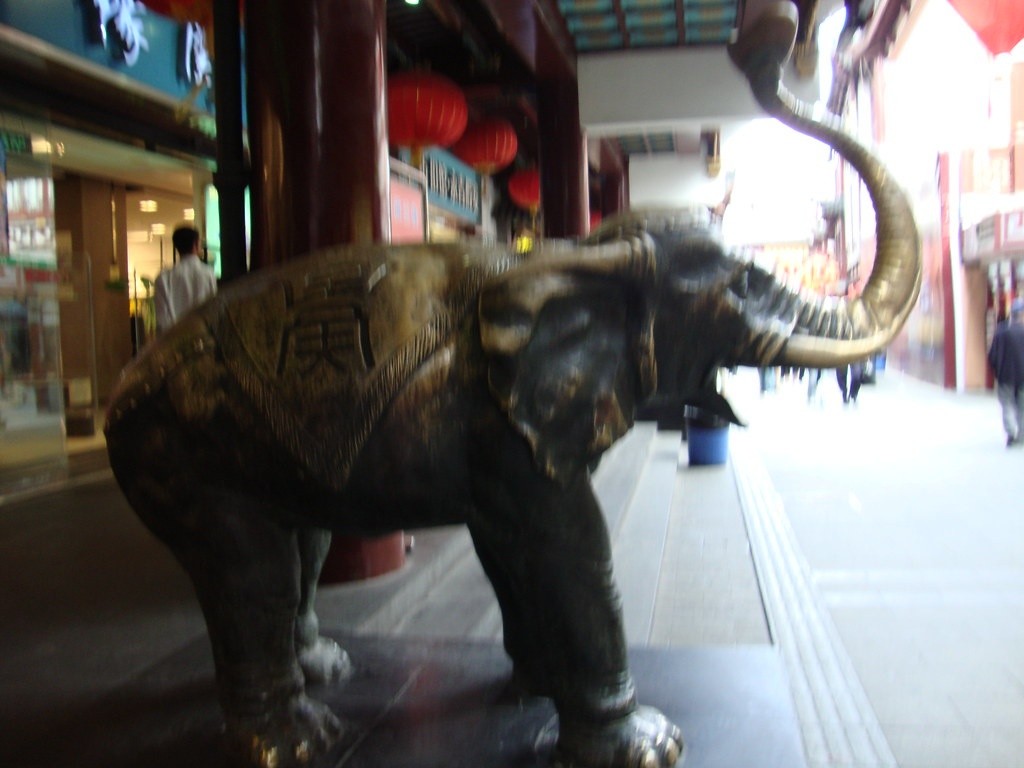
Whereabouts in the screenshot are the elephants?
[101,0,924,767]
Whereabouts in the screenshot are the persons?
[759,366,822,402]
[835,361,862,403]
[987,299,1024,446]
[154,226,218,338]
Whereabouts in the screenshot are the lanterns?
[590,207,602,229]
[510,167,540,215]
[450,115,518,178]
[387,68,469,163]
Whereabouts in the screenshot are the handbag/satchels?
[859,357,875,383]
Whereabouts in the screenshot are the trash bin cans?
[684,404,730,466]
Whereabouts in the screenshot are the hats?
[1011,300,1024,313]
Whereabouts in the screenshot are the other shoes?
[1007,436,1014,447]
